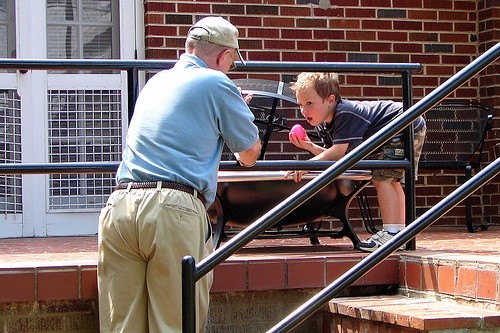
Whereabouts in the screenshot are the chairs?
[409,99,497,232]
[219,103,282,162]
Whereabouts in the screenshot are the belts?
[115,180,206,205]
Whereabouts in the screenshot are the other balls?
[291,124,305,139]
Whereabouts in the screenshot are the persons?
[285,71,426,252]
[97,16,261,332]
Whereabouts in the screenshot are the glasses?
[221,47,238,70]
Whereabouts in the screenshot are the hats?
[187,16,247,66]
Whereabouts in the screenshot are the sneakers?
[356,230,406,253]
